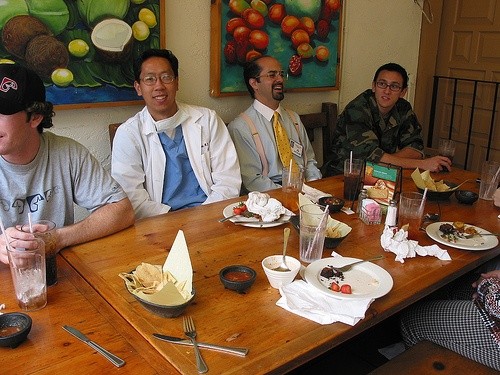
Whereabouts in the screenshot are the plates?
[425,222,498,250]
[304,257,394,300]
[222,201,290,228]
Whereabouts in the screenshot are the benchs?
[108,101,338,171]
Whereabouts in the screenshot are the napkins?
[273,279,375,326]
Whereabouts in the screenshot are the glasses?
[139,74,176,86]
[374,80,404,92]
[255,71,288,81]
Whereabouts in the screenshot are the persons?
[0,58,135,265]
[227,56,322,194]
[110,48,242,220]
[319,63,453,177]
[398,262,500,369]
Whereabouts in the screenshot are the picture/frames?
[0,0,166,109]
[209,0,345,96]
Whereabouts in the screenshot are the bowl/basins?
[416,180,459,200]
[125,269,196,318]
[319,196,344,214]
[0,311,32,348]
[219,266,257,294]
[455,190,478,205]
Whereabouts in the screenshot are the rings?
[21,224,25,232]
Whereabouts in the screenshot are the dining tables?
[59,165,500,375]
[1,253,182,375]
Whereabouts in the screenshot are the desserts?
[366,179,388,199]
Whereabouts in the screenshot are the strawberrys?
[329,282,352,294]
[233,202,246,215]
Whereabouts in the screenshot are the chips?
[324,226,342,238]
[117,262,186,296]
[422,178,450,192]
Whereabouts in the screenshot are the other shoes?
[377,342,405,360]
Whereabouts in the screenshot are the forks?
[460,233,499,239]
[328,254,385,273]
[183,316,208,373]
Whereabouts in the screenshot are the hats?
[0,67,46,115]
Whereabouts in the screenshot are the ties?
[273,111,306,184]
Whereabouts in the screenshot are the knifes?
[62,325,126,368]
[234,220,289,224]
[152,332,248,357]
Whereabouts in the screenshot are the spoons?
[275,228,291,272]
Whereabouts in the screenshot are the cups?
[21,220,58,287]
[6,237,47,311]
[300,204,329,263]
[262,255,302,289]
[397,191,426,240]
[281,166,303,216]
[343,159,364,202]
[478,161,500,201]
[439,139,456,175]
[291,217,349,249]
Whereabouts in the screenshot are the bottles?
[384,200,397,227]
[356,189,367,214]
